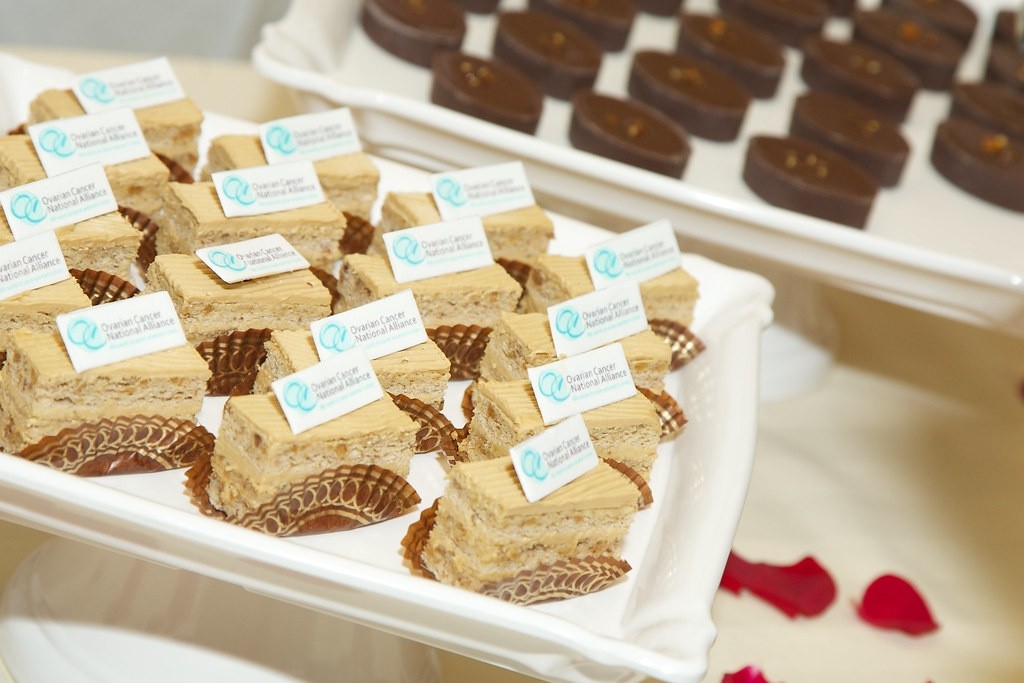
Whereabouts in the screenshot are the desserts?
[0,80,708,609]
[357,0,1024,232]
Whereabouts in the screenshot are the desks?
[0,46,1024,683]
